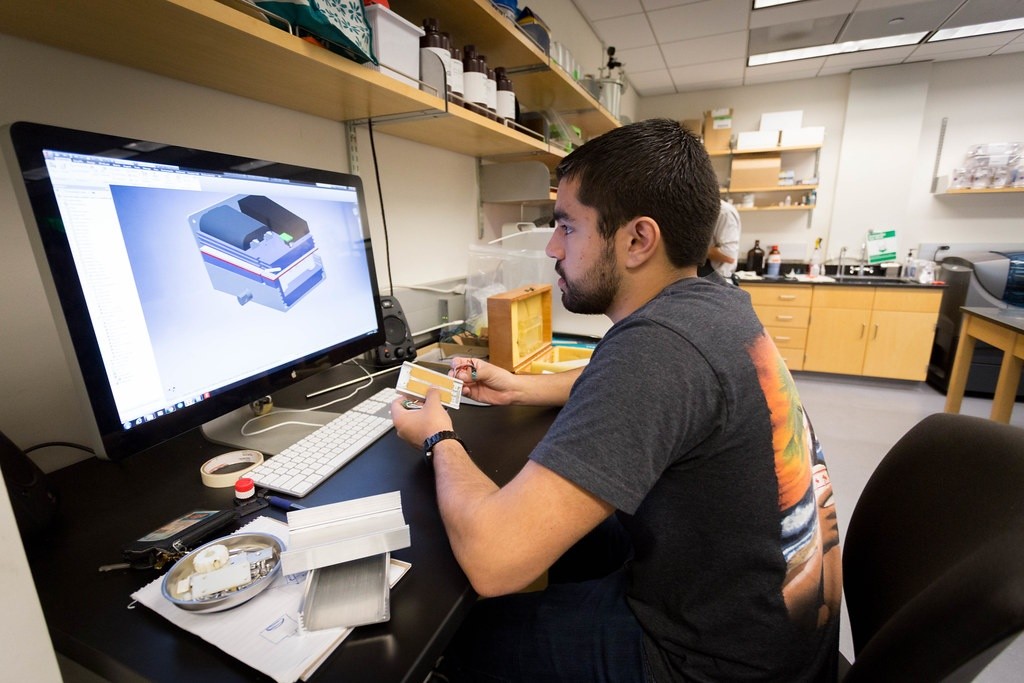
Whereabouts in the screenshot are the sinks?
[832,276,920,285]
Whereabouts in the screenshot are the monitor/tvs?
[10,120,388,467]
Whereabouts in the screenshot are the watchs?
[423,431,469,473]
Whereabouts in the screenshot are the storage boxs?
[779,126,826,147]
[728,154,781,189]
[866,229,898,266]
[413,342,489,375]
[677,120,702,137]
[737,130,780,149]
[758,110,804,131]
[360,4,426,90]
[702,108,734,150]
[487,284,594,375]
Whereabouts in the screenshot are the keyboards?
[237,388,403,497]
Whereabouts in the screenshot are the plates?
[161,532,286,613]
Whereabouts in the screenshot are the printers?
[926,249,1024,403]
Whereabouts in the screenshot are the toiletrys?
[808,236,823,279]
[900,248,918,279]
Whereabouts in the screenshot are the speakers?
[372,295,417,366]
[0,431,59,524]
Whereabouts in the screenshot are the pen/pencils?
[264,495,308,510]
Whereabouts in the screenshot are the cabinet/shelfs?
[803,285,944,385]
[738,283,813,371]
[0,0,622,177]
[708,145,824,229]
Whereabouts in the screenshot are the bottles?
[801,193,808,205]
[785,196,792,206]
[778,170,795,186]
[807,192,817,205]
[747,240,765,276]
[766,246,781,276]
[419,18,515,129]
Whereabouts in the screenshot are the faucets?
[836,246,848,276]
[849,242,874,276]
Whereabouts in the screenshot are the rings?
[472,366,477,382]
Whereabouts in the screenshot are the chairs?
[809,413,1024,683]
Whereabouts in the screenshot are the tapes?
[200,449,264,488]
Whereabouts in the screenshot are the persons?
[698,199,741,285]
[390,117,843,683]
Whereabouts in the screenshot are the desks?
[15,332,598,683]
[941,305,1024,425]
